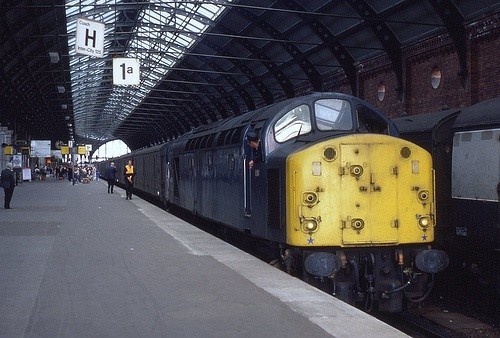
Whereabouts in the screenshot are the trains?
[390,97,500,326]
[93,93,450,321]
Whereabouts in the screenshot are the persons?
[123,159,136,200]
[245,132,262,169]
[0,162,16,210]
[34,163,101,184]
[105,161,118,194]
